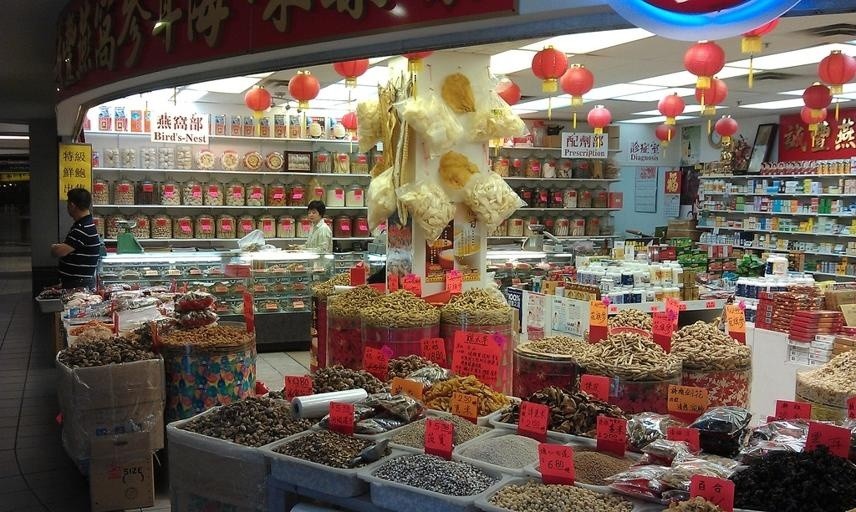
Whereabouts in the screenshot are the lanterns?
[531,44,568,92]
[695,77,727,116]
[288,69,320,109]
[817,49,856,97]
[244,84,272,120]
[558,63,594,108]
[739,18,779,56]
[658,94,686,125]
[333,59,370,88]
[802,81,833,118]
[342,112,357,130]
[587,104,612,135]
[800,106,827,131]
[683,39,726,89]
[714,117,738,144]
[496,76,521,106]
[656,124,676,148]
[401,52,433,73]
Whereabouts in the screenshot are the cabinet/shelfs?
[83,129,623,243]
[507,280,726,325]
[694,174,856,278]
[96,251,547,344]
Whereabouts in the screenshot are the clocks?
[708,125,723,149]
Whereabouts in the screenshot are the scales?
[115,220,144,254]
[522,224,544,251]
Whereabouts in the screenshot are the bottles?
[733,300,757,323]
[525,154,541,178]
[92,176,109,205]
[106,209,126,239]
[128,208,150,240]
[245,177,265,206]
[151,211,172,239]
[158,146,174,169]
[266,177,286,206]
[314,146,331,174]
[562,184,577,208]
[296,214,312,238]
[509,153,525,177]
[305,178,326,206]
[286,179,305,207]
[493,155,509,177]
[547,184,562,208]
[765,255,775,276]
[773,254,788,275]
[216,211,236,238]
[175,145,193,170]
[532,184,548,208]
[182,176,202,206]
[113,176,135,205]
[735,273,815,301]
[578,184,591,208]
[332,150,349,174]
[523,213,539,237]
[334,213,352,238]
[365,184,370,207]
[554,212,569,236]
[203,175,224,205]
[573,158,590,178]
[257,212,276,238]
[353,213,369,236]
[140,146,157,169]
[102,147,119,168]
[541,213,553,236]
[172,215,193,239]
[345,181,365,207]
[492,220,507,237]
[542,154,556,178]
[569,213,585,236]
[136,175,158,204]
[225,177,245,206]
[350,147,369,175]
[161,175,181,205]
[276,214,295,238]
[592,185,606,207]
[93,209,105,239]
[326,180,345,207]
[599,211,614,236]
[508,213,524,237]
[194,213,215,239]
[237,212,256,238]
[577,261,684,304]
[557,157,573,179]
[121,147,136,169]
[515,183,532,208]
[585,212,599,236]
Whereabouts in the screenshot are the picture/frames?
[747,123,775,174]
[283,150,313,172]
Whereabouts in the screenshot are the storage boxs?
[89,451,154,512]
[603,126,620,149]
[489,409,597,444]
[428,395,521,426]
[667,219,694,229]
[357,452,507,512]
[167,405,267,512]
[376,420,494,453]
[312,407,436,441]
[36,297,61,312]
[453,428,562,477]
[544,135,561,147]
[524,440,643,494]
[259,429,407,498]
[667,229,698,240]
[57,348,164,459]
[474,476,643,512]
[606,192,622,207]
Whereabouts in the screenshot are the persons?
[302,200,333,253]
[49,188,100,286]
